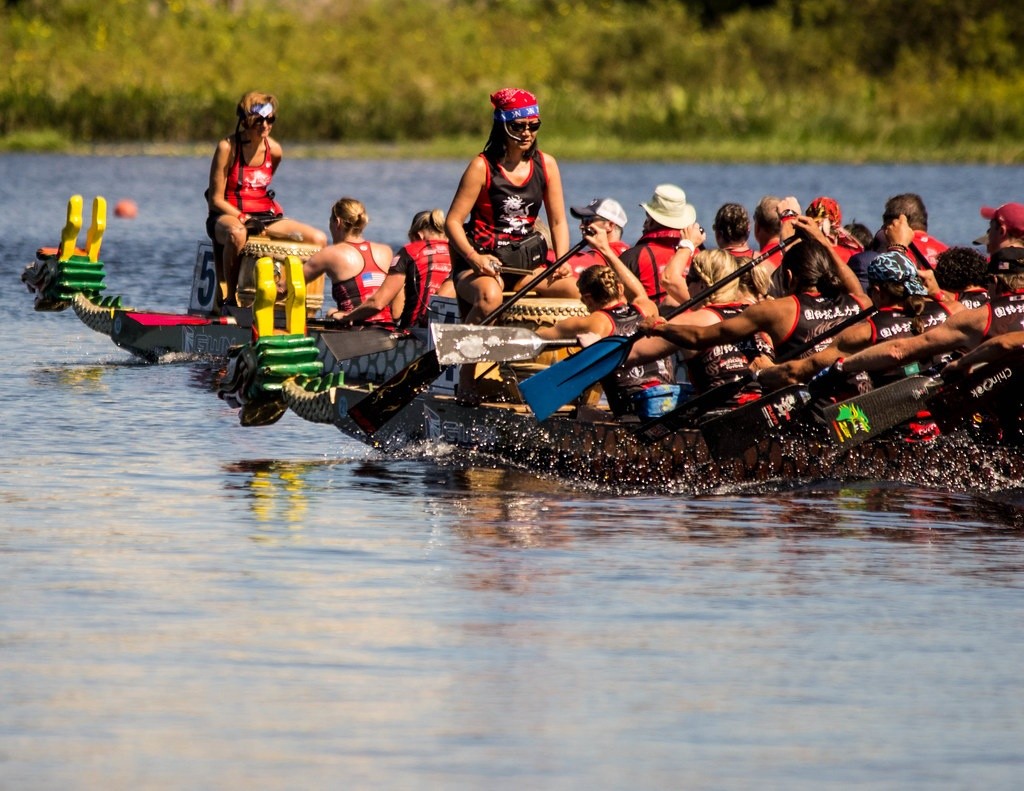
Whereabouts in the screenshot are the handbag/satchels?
[496,231,548,271]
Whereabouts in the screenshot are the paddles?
[698,370,837,465]
[321,325,434,364]
[630,304,883,448]
[343,227,597,438]
[518,225,808,423]
[429,322,582,368]
[823,350,1024,454]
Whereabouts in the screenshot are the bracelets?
[886,244,908,254]
[646,321,665,339]
[464,246,474,259]
[675,239,694,257]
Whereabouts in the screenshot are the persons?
[531,183,1024,445]
[204,90,328,316]
[444,88,627,407]
[325,209,456,330]
[234,197,405,332]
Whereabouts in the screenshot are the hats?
[639,184,697,229]
[570,198,627,227]
[981,202,1024,231]
[972,229,990,245]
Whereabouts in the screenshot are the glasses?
[582,216,605,226]
[509,119,542,132]
[253,116,276,124]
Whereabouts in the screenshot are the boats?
[22,194,1024,527]
